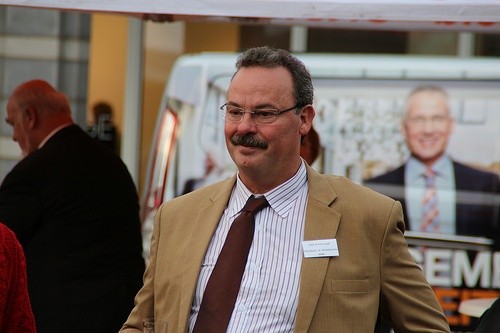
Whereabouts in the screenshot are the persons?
[118,46,450,332]
[0,222,35,333]
[361,88,496,237]
[0,79,146,333]
[87,100,120,158]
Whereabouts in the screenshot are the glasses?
[220,104,296,124]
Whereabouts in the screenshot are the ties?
[192,195,274,333]
[420,170,440,233]
